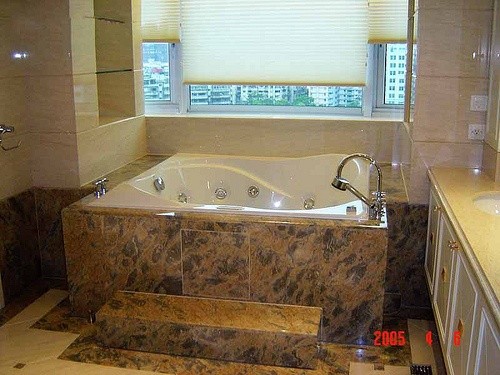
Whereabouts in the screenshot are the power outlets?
[468,124,485,140]
[470,95,487,112]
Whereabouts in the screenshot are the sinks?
[469,188,500,218]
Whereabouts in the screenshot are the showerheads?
[330,176,381,218]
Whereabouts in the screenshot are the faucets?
[332,151,383,217]
[0,124,15,144]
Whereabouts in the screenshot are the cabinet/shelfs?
[430,212,460,351]
[444,253,483,375]
[423,186,442,300]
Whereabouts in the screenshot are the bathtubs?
[87,150,373,222]
